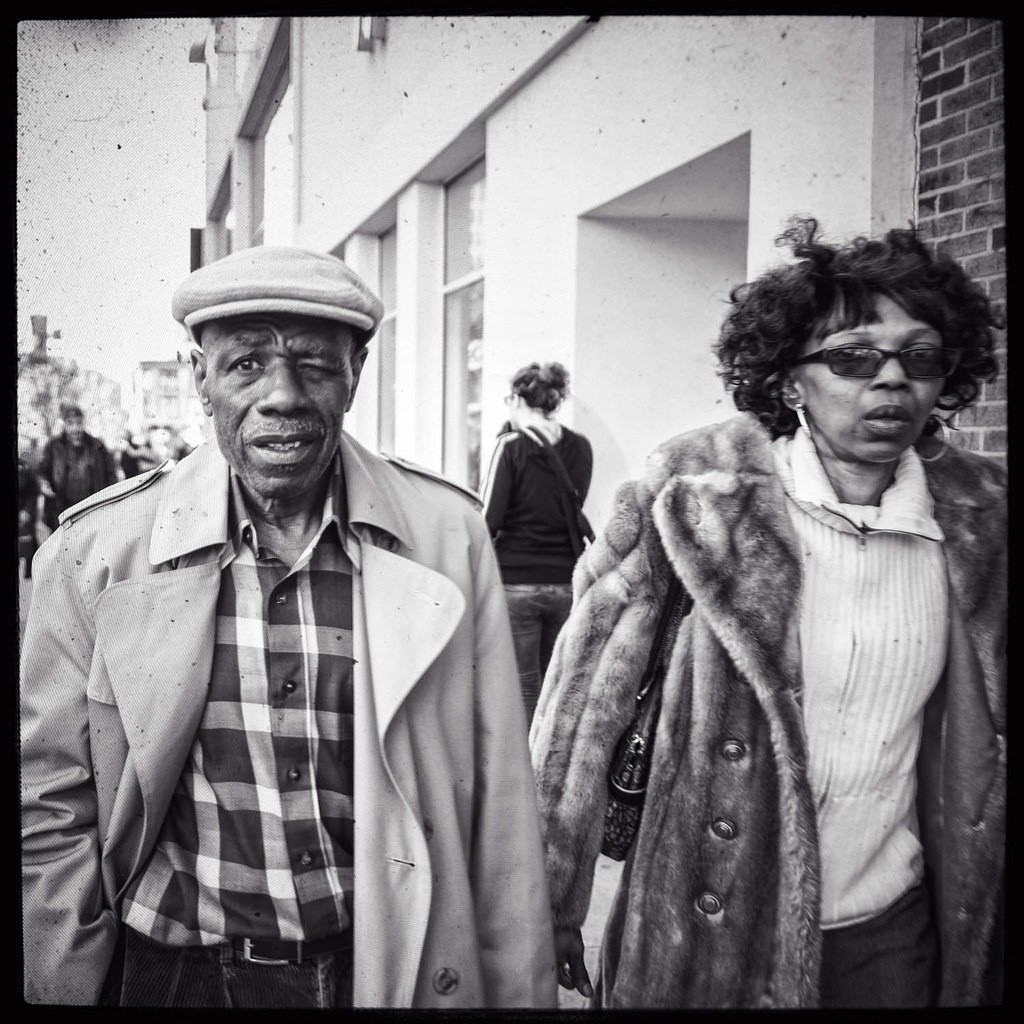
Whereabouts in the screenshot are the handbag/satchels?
[595,577,687,861]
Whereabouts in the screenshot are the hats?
[171,243,383,345]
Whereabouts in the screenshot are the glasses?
[778,346,962,381]
[505,394,522,405]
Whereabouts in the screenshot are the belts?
[213,935,353,965]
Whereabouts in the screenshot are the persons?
[482,364,592,730]
[18,405,194,579]
[526,216,1008,1005]
[18,245,559,1010]
